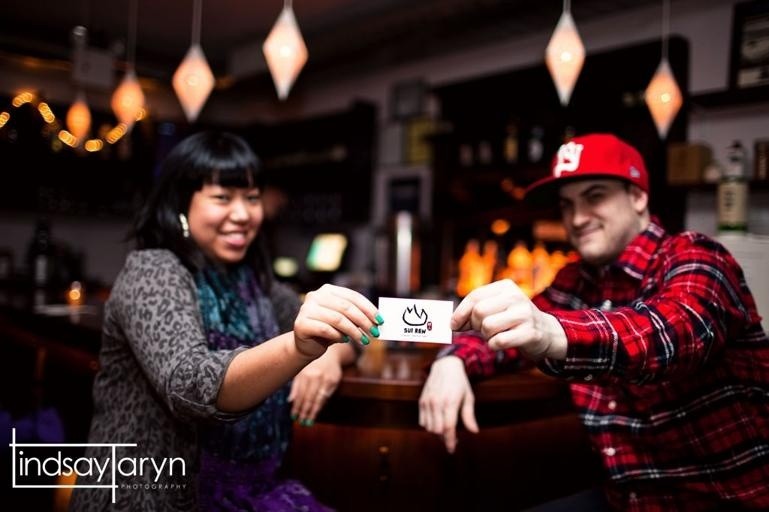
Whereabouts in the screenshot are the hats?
[522,134,650,215]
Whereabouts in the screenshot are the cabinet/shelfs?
[669,83,768,196]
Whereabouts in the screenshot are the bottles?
[716,153,749,234]
[449,237,583,299]
[26,211,53,286]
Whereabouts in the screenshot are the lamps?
[542,1,684,142]
[65,1,311,138]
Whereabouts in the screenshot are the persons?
[416,130,769,511]
[62,125,386,512]
